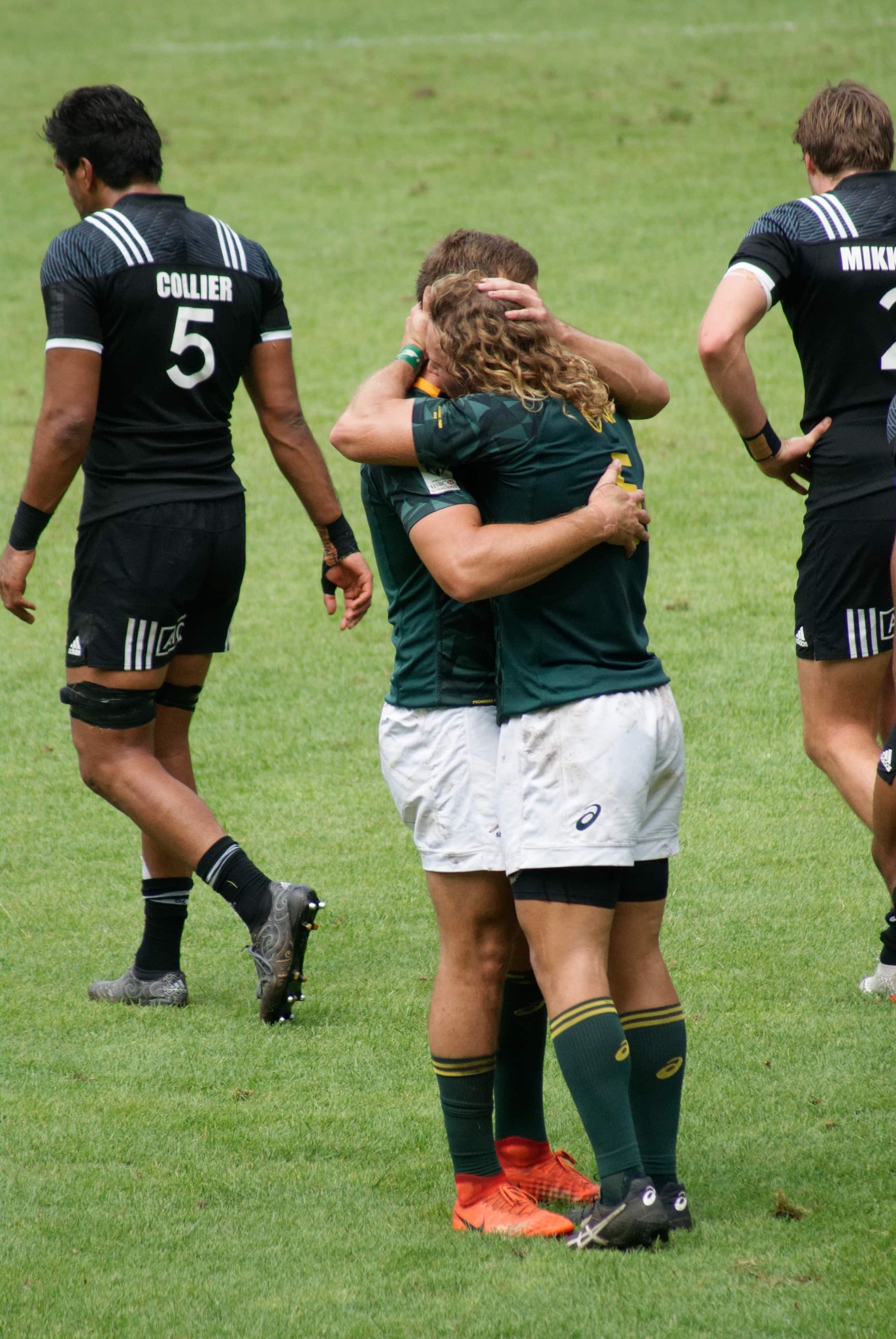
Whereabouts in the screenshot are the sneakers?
[565,1173,692,1233]
[452,1169,573,1239]
[561,1176,670,1253]
[88,966,188,1009]
[240,881,326,1025]
[859,958,896,1002]
[493,1136,601,1206]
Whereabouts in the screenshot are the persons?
[0,82,374,1023]
[327,276,698,1251]
[696,82,896,1003]
[357,230,677,1234]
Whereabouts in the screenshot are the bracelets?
[7,499,54,551]
[738,418,782,463]
[394,343,426,377]
